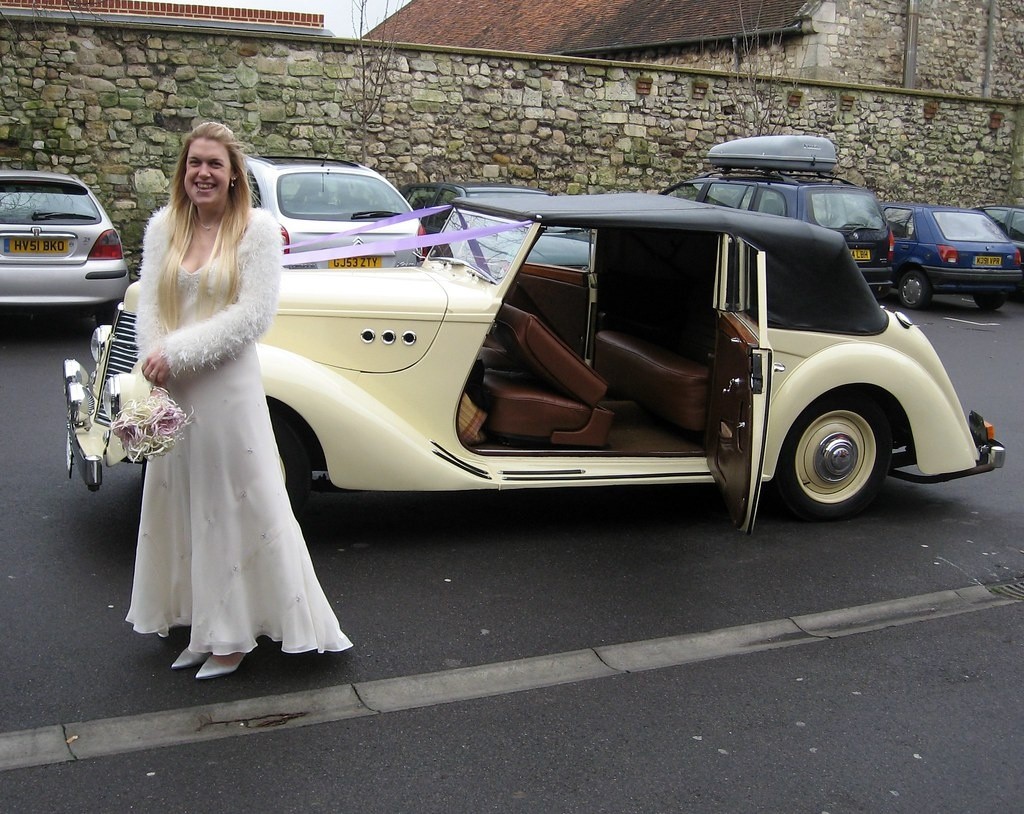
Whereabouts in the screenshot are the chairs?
[306,181,339,213]
[760,197,784,217]
[717,195,735,208]
[477,280,615,448]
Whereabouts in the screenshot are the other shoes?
[171,647,211,668]
[196,653,246,680]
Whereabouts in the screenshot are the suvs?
[242,150,430,269]
[660,133,896,302]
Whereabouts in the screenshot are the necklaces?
[200,222,213,231]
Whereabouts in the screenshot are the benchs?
[594,258,712,433]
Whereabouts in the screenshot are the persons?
[125,125,354,679]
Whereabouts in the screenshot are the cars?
[830,203,1024,310]
[969,205,1024,299]
[61,191,1008,541]
[0,167,128,329]
[396,179,595,272]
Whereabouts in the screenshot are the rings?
[151,373,156,377]
